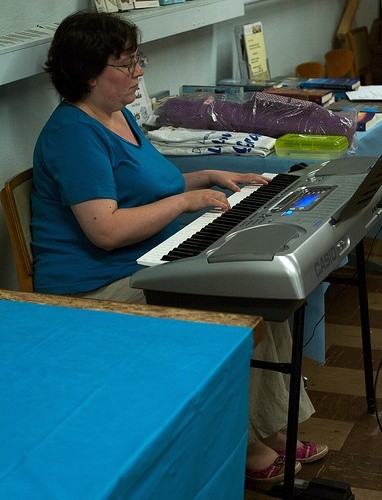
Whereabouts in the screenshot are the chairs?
[0,165,33,296]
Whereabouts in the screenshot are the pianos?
[129,155,381,500]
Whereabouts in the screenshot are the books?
[176,79,362,107]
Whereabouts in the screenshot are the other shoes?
[273,440,329,463]
[245,453,302,484]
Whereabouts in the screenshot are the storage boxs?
[275,134,348,160]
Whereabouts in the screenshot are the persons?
[31,13,330,480]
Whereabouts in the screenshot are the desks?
[142,237,377,497]
[163,121,382,365]
[0,290,264,500]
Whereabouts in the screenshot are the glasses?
[106,55,145,75]
